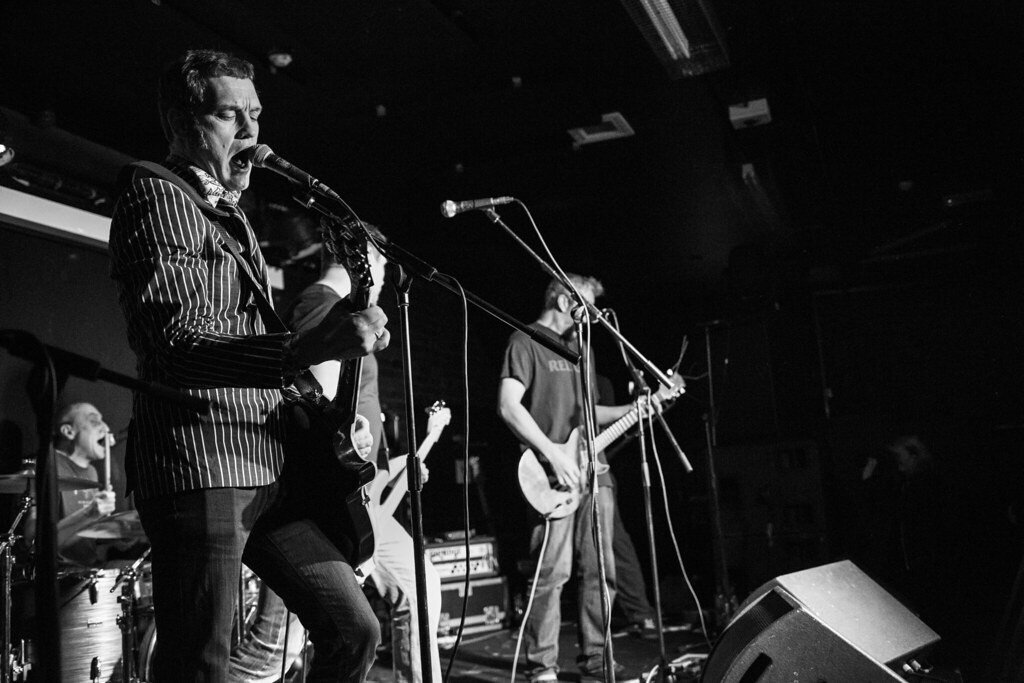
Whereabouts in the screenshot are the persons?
[496,272,663,683]
[24,401,116,575]
[108,49,445,683]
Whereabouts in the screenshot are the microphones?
[107,428,128,447]
[248,143,340,202]
[440,196,515,218]
[590,308,611,323]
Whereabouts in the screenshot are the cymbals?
[73,508,148,540]
[0,457,102,492]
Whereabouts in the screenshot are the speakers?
[698,556,945,683]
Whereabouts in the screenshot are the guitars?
[515,368,687,521]
[282,207,376,572]
[352,398,452,584]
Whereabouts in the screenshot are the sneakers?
[581,660,642,683]
[640,618,660,640]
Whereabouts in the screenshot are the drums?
[54,558,157,683]
